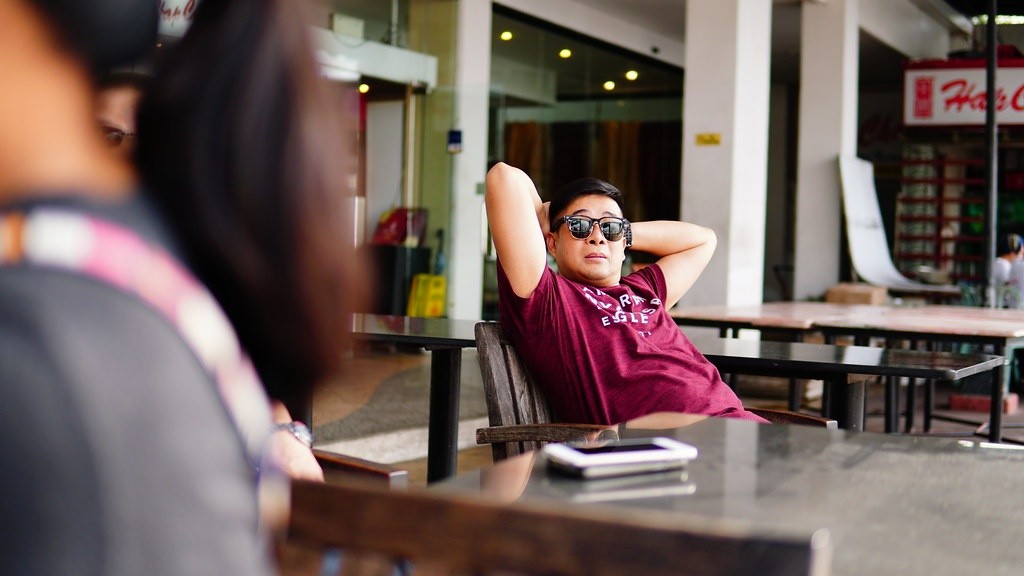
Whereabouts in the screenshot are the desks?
[888,284,962,304]
[685,338,1006,443]
[419,411,1024,575]
[816,306,1024,447]
[665,299,838,411]
[286,312,478,483]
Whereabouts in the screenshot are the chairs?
[475,321,840,463]
[269,450,833,576]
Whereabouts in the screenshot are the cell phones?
[543,436,698,474]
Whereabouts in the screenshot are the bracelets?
[625,226,632,252]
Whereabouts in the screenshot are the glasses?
[550,215,631,243]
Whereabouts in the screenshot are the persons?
[994,233,1024,411]
[483,163,772,429]
[0,2,331,576]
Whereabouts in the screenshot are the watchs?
[245,418,314,473]
